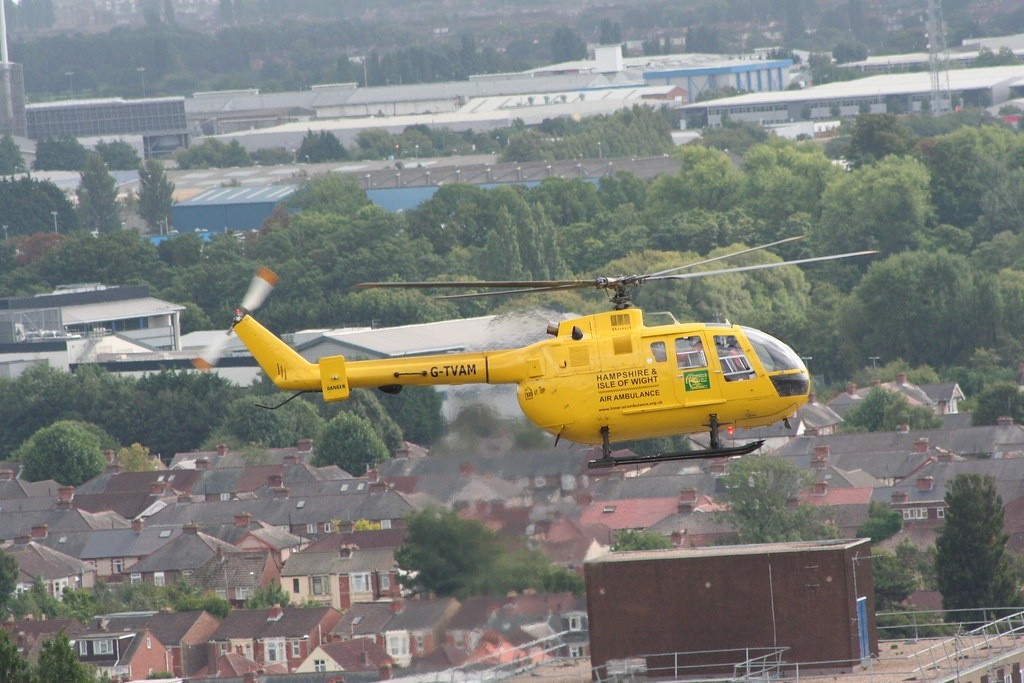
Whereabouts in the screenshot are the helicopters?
[233,231,884,471]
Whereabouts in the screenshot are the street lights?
[138,67,148,99]
[51,211,58,232]
[3,225,8,239]
[64,71,75,99]
[366,134,613,184]
[292,149,296,164]
[305,155,309,164]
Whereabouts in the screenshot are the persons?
[683,335,751,378]
